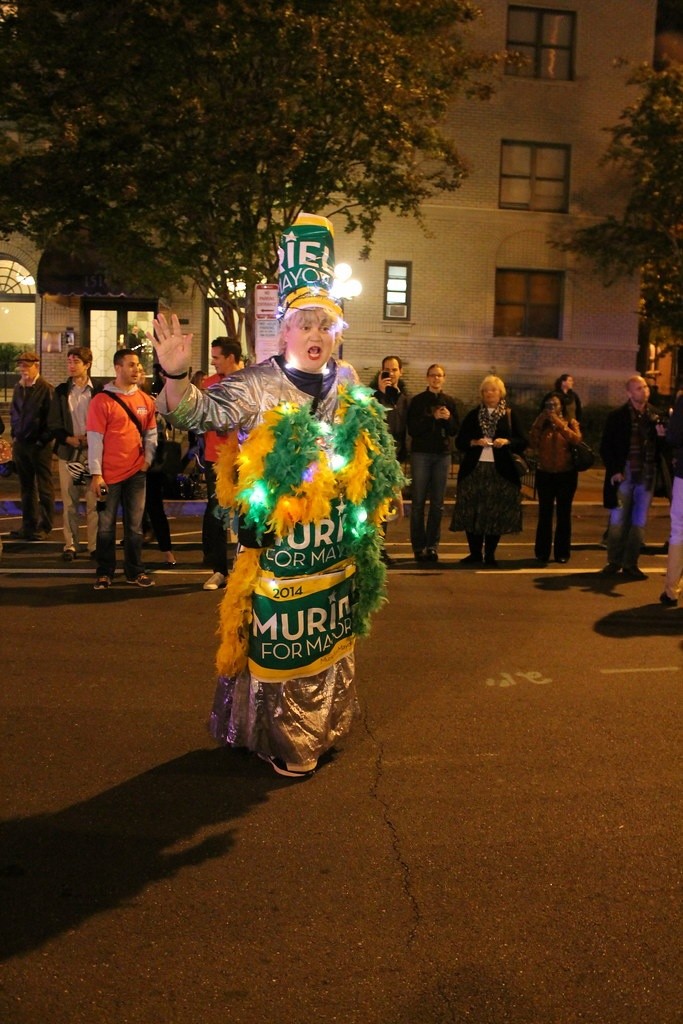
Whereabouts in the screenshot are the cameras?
[100,487,106,495]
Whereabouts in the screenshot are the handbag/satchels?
[511,452,530,476]
[152,439,182,473]
[572,441,595,471]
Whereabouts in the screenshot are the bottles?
[97,487,107,512]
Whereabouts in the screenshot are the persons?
[178,371,208,479]
[128,325,141,355]
[659,390,683,606]
[526,391,582,562]
[145,413,177,566]
[145,226,404,776]
[47,346,98,562]
[407,364,461,561]
[449,375,525,566]
[85,348,158,590]
[369,355,412,564]
[9,353,55,540]
[200,337,243,589]
[538,374,582,422]
[600,376,670,579]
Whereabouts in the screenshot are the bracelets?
[162,370,188,380]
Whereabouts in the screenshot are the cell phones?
[382,372,390,379]
[544,402,555,410]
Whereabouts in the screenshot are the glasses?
[428,374,444,378]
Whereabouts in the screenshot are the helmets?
[66,459,92,483]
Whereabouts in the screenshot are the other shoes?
[90,550,97,558]
[30,529,48,540]
[660,590,678,607]
[168,557,177,568]
[9,527,32,538]
[63,550,77,561]
[484,554,495,562]
[622,568,648,581]
[426,549,438,561]
[538,552,550,562]
[414,552,427,561]
[461,555,483,563]
[554,551,570,563]
[601,563,622,576]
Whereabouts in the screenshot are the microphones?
[651,413,661,425]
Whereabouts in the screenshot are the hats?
[276,212,343,321]
[16,352,40,367]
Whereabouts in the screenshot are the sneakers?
[203,572,230,590]
[258,752,318,778]
[127,573,156,587]
[94,575,112,590]
